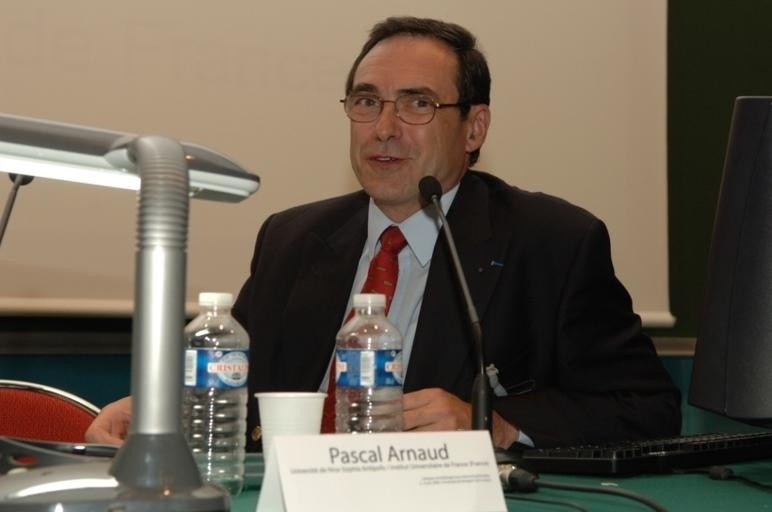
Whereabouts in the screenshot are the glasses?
[340,94,460,126]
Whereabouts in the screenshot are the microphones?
[1,174,33,241]
[418,175,494,438]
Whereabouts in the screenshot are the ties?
[321,225,407,432]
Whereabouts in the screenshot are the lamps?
[0,113,261,512]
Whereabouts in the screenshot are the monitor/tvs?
[688,95,772,489]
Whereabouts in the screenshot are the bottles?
[334,292,405,434]
[184,291,248,494]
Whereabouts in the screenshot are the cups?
[255,392,326,437]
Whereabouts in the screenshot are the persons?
[84,16,682,452]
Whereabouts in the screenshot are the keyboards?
[521,433,768,477]
